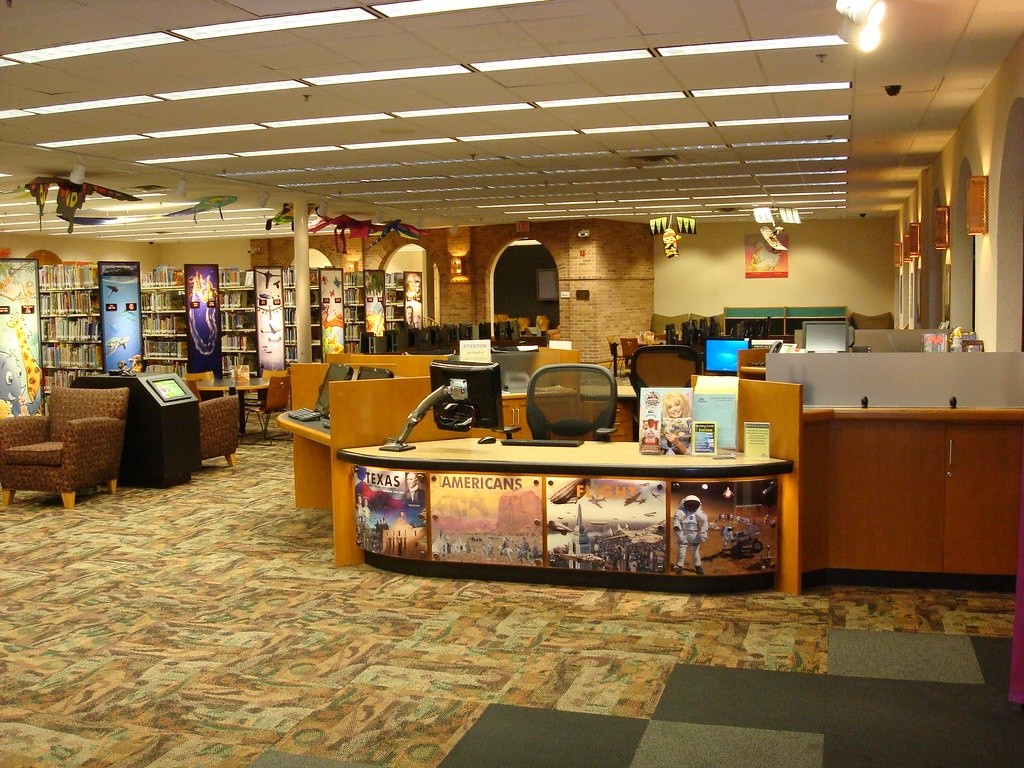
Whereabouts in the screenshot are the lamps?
[894,176,990,271]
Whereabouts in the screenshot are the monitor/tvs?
[384,320,521,352]
[316,361,354,418]
[360,332,374,353]
[378,360,503,453]
[665,317,721,348]
[356,366,394,381]
[152,380,187,399]
[703,337,751,377]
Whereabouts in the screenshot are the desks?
[194,377,271,437]
[340,438,795,594]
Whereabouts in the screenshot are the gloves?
[673,526,680,532]
[700,537,706,542]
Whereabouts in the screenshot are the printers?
[765,321,872,354]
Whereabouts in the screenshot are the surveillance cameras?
[885,85,901,96]
[860,214,865,217]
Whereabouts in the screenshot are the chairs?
[629,345,698,391]
[181,380,239,466]
[606,330,667,377]
[527,364,617,442]
[0,385,129,509]
[185,365,292,446]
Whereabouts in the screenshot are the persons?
[672,495,751,574]
[401,472,425,508]
[642,428,659,445]
[660,393,691,454]
[927,334,942,352]
[465,536,541,566]
[357,496,376,552]
[405,306,415,328]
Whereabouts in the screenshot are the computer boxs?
[369,325,481,354]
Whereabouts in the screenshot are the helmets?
[682,495,701,512]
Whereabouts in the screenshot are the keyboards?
[288,408,322,422]
[501,439,584,447]
[323,420,331,428]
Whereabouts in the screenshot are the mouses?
[478,436,496,444]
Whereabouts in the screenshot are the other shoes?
[695,565,704,573]
[676,564,683,575]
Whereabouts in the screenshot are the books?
[219,266,258,400]
[385,273,404,331]
[343,272,364,353]
[282,267,298,369]
[141,265,187,381]
[309,270,322,362]
[38,264,101,417]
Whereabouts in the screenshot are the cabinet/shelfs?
[37,260,422,416]
